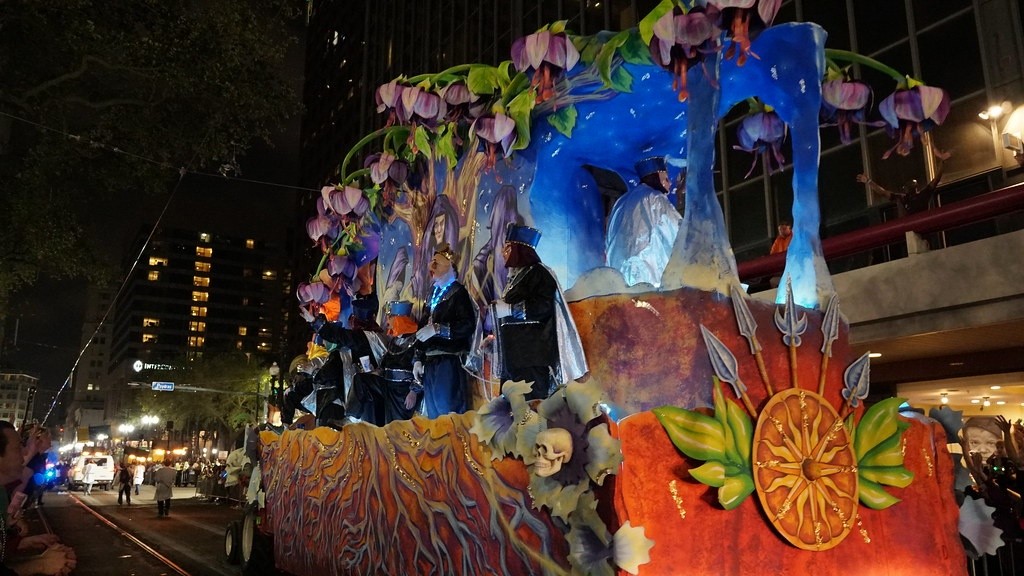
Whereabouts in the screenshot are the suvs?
[68,454,114,491]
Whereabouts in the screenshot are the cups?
[359,356,372,373]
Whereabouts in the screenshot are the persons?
[82,460,97,496]
[284,297,391,426]
[769,220,792,287]
[55,461,71,485]
[411,249,475,418]
[154,461,177,517]
[494,224,589,399]
[856,148,953,250]
[334,300,422,424]
[959,414,1024,576]
[962,416,1002,465]
[602,157,683,286]
[115,460,146,506]
[0,419,77,576]
[146,458,211,487]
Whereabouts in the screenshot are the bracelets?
[867,179,871,183]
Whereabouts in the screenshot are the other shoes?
[87,490,91,495]
[165,514,171,517]
[118,499,123,504]
[127,500,131,506]
[84,489,88,496]
[159,511,164,515]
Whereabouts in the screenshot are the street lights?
[118,424,133,445]
[143,415,159,449]
